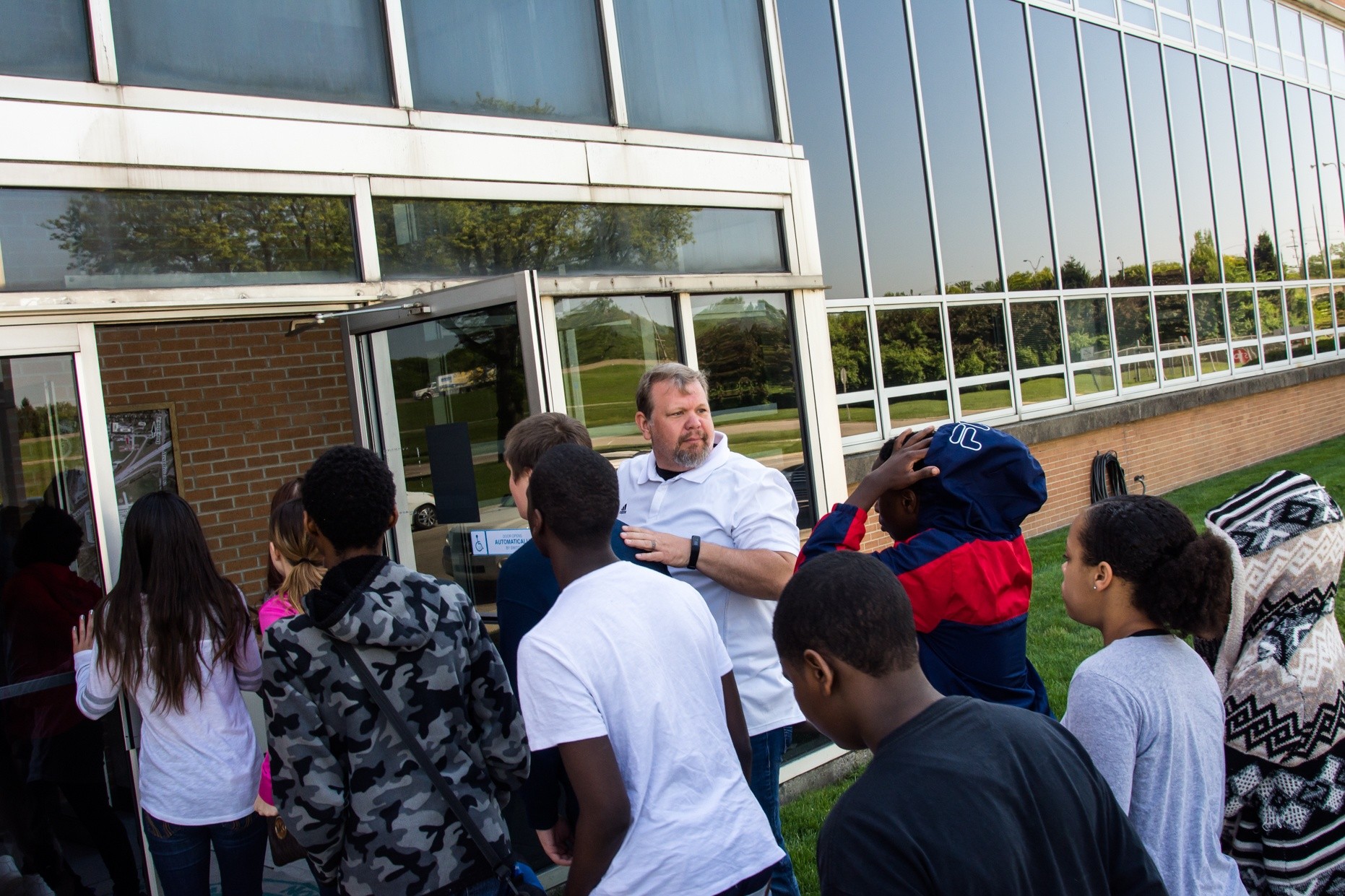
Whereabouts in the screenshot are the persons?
[251,446,531,896]
[0,469,140,896]
[794,425,1057,719]
[72,490,260,896]
[496,360,801,896]
[1061,492,1250,896]
[772,551,1170,896]
[1193,470,1345,896]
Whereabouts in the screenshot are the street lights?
[1022,256,1045,278]
[1116,256,1125,284]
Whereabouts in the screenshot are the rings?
[650,539,656,552]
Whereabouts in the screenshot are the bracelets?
[687,535,701,569]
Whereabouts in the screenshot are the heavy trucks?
[412,373,498,400]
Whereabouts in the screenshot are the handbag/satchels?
[495,861,546,896]
